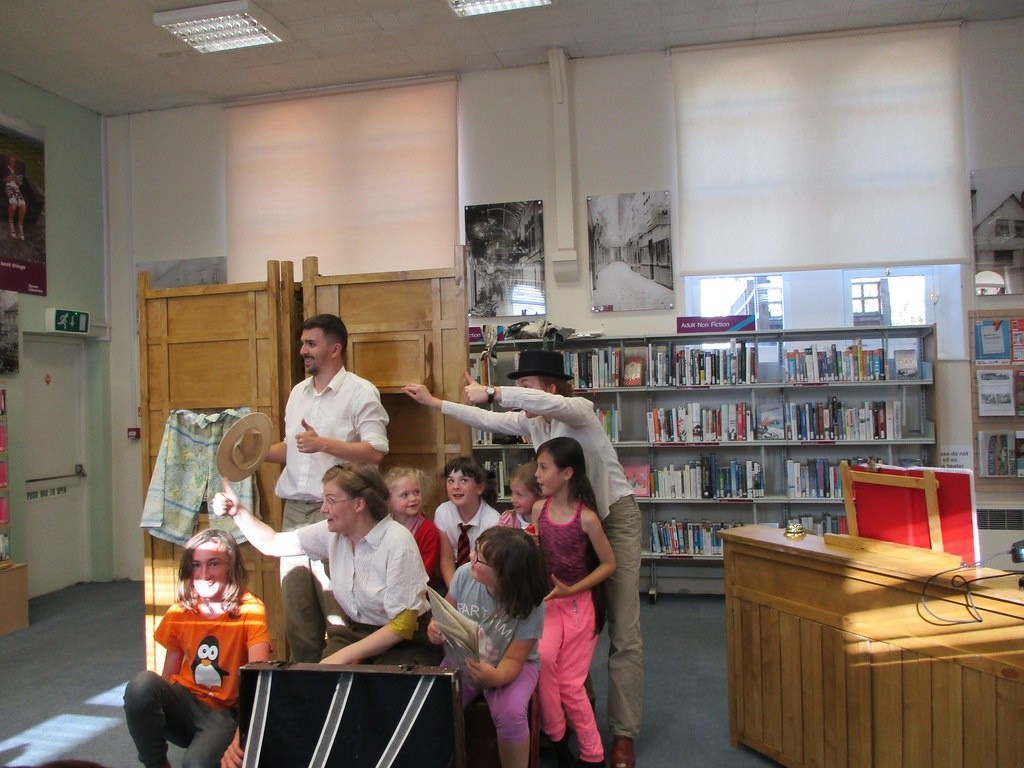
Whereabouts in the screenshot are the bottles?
[527,525,545,568]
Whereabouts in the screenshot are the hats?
[507,349,574,380]
[216,412,273,482]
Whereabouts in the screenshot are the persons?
[498,459,547,528]
[433,456,500,570]
[3,154,27,240]
[400,350,643,768]
[263,313,389,664]
[383,465,441,579]
[124,527,269,768]
[530,437,617,768]
[213,463,443,667]
[427,526,550,768]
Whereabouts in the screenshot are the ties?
[457,523,473,566]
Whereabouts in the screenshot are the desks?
[0,561,29,639]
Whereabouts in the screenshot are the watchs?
[486,383,496,404]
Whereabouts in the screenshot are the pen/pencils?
[440,632,453,648]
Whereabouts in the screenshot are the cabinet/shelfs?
[469,325,935,605]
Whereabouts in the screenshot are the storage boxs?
[677,315,755,333]
[469,326,504,342]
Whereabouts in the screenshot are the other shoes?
[613,735,634,768]
[553,725,606,768]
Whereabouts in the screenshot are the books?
[621,452,766,499]
[787,512,850,536]
[786,338,886,384]
[646,395,902,442]
[644,338,756,387]
[475,403,617,446]
[786,457,926,499]
[652,518,752,555]
[469,346,644,389]
[974,316,1024,478]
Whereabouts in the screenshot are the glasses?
[321,493,365,505]
